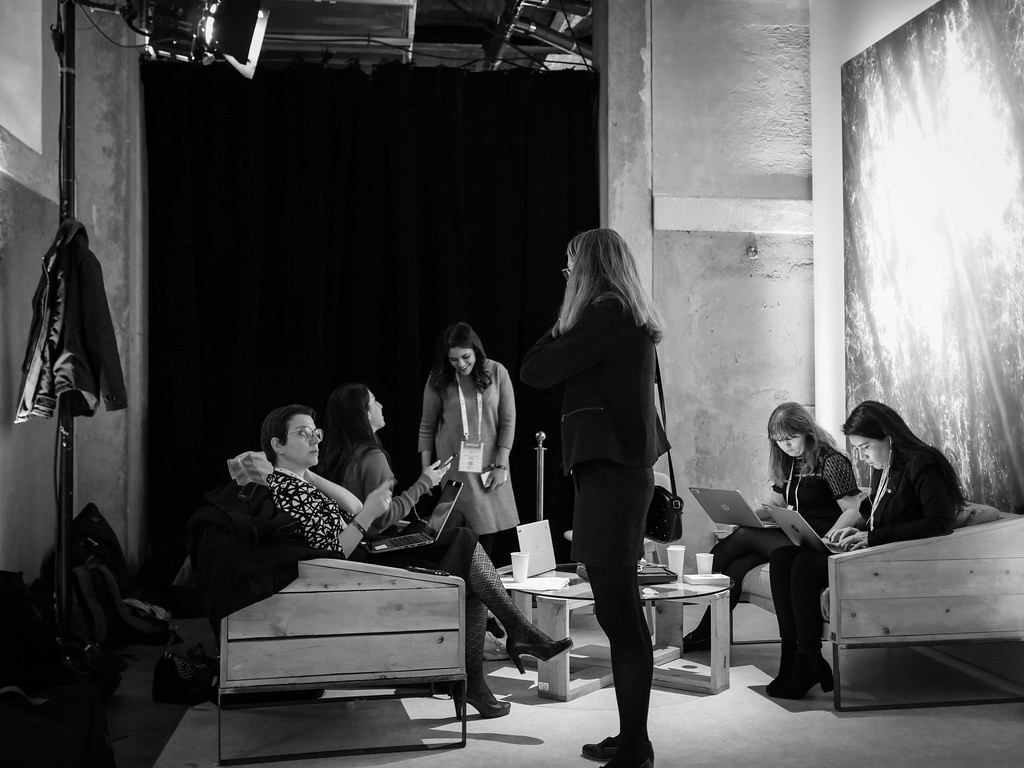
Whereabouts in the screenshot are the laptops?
[688,487,780,528]
[761,503,867,554]
[364,480,464,554]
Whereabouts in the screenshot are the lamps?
[113,0,271,80]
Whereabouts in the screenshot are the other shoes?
[682,624,733,651]
[581,736,656,761]
[599,756,653,768]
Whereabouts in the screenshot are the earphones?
[889,436,892,444]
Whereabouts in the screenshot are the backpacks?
[0,497,174,768]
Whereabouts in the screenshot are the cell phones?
[480,470,507,488]
[439,453,458,470]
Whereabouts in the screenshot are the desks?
[512,562,735,702]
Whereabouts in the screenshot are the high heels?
[450,681,511,719]
[508,636,573,675]
[767,649,835,700]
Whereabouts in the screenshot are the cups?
[510,552,529,583]
[695,553,713,574]
[666,545,686,576]
[670,577,684,597]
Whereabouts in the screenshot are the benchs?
[218,451,466,766]
[713,487,1024,712]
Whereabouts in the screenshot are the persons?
[682,402,863,654]
[419,322,520,635]
[259,405,574,720]
[765,399,965,700]
[315,385,451,539]
[520,229,672,768]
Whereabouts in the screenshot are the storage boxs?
[683,574,730,584]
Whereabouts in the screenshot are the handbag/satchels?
[152,641,221,704]
[643,485,682,543]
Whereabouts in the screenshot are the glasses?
[561,267,574,280]
[284,426,324,442]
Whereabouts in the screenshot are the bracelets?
[351,520,366,536]
[494,465,507,470]
